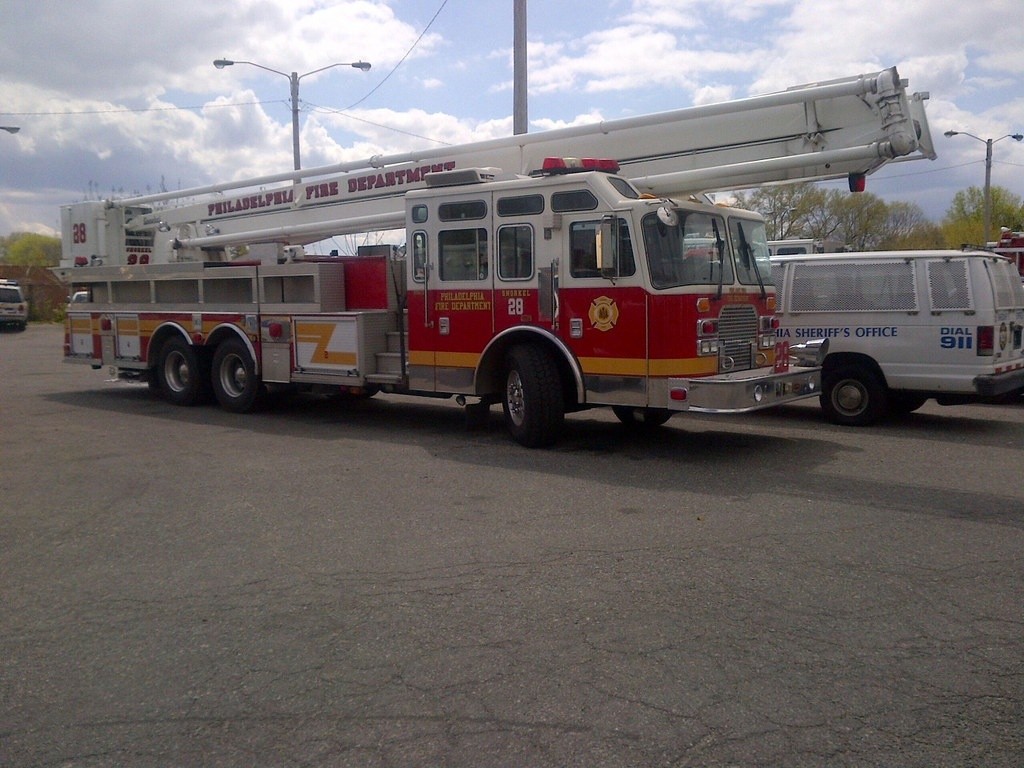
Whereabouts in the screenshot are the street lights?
[763,207,798,239]
[944,131,1023,243]
[213,59,371,183]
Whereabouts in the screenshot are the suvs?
[0,281,30,328]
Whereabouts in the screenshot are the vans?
[683,237,817,255]
[702,250,1024,421]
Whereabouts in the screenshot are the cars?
[65,291,91,304]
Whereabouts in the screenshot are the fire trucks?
[42,66,939,444]
[962,225,1024,285]
